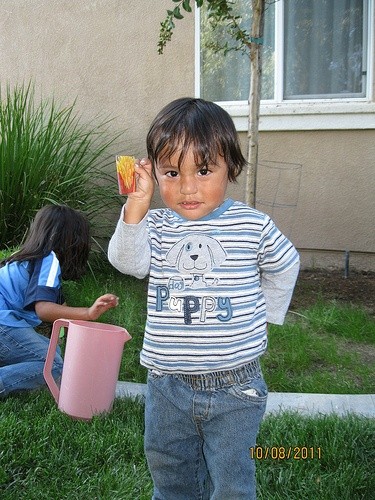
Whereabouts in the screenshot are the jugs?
[41,318,132,421]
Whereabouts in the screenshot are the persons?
[107,97,300,500]
[0,203,119,401]
